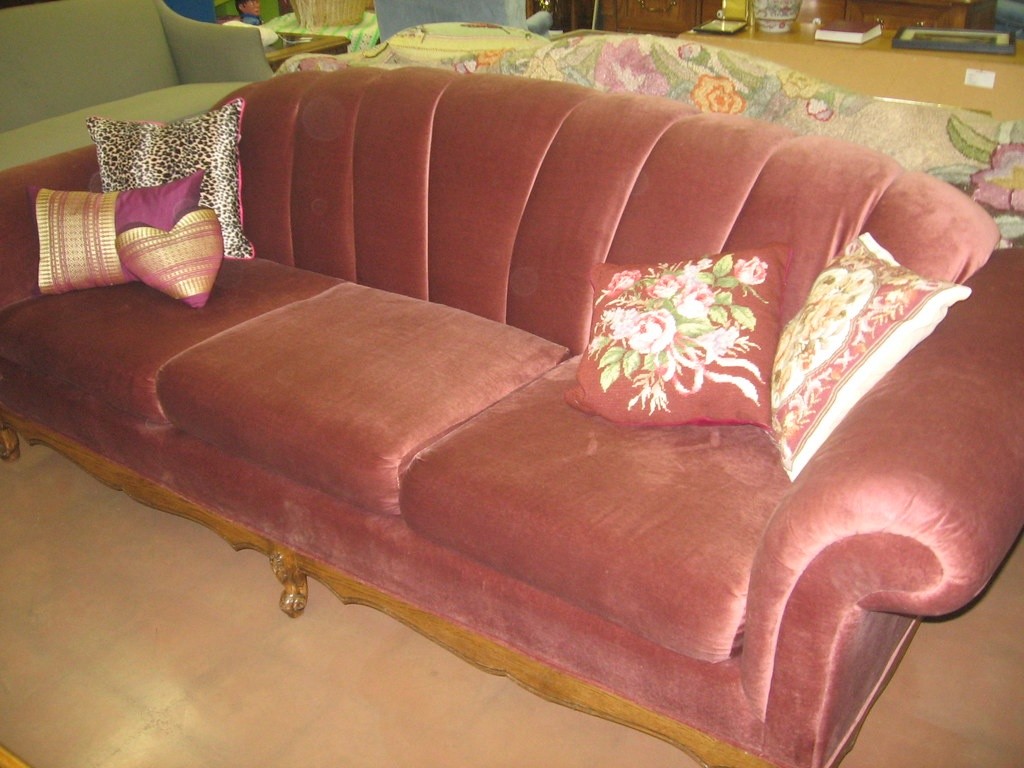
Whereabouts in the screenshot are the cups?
[752,0,802,33]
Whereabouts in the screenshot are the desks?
[263,12,381,54]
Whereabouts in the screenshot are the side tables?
[263,31,351,73]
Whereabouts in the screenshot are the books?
[814,17,882,45]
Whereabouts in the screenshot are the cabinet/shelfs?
[524,0,997,38]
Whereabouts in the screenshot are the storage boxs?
[678,16,1023,120]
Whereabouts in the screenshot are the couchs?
[0,68,1024,768]
[278,33,1024,252]
[0,0,272,172]
[374,0,554,44]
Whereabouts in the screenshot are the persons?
[235,0,264,26]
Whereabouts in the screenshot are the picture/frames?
[693,17,749,34]
[892,25,1016,55]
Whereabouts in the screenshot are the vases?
[751,0,802,31]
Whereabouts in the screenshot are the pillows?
[116,205,224,308]
[386,22,550,62]
[27,169,207,294]
[564,244,791,430]
[762,232,971,482]
[86,97,256,261]
